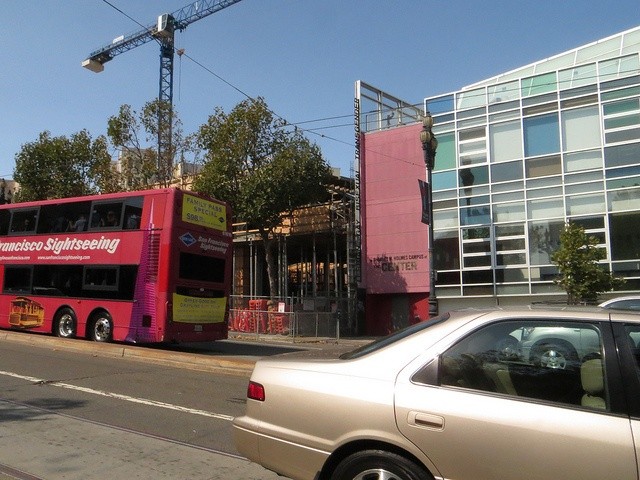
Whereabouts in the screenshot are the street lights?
[419,110,440,317]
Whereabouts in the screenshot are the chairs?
[580,358,606,410]
